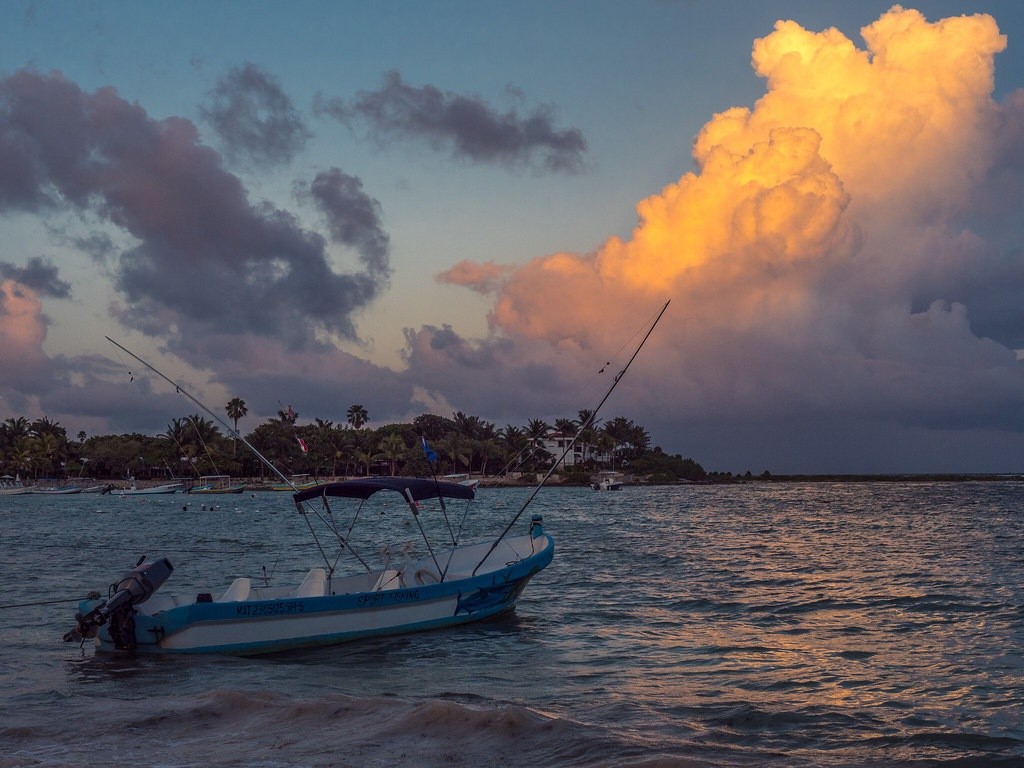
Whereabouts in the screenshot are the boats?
[0,485,37,494]
[109,483,183,494]
[188,485,246,493]
[272,479,324,491]
[32,486,82,494]
[61,477,554,659]
[590,472,624,490]
